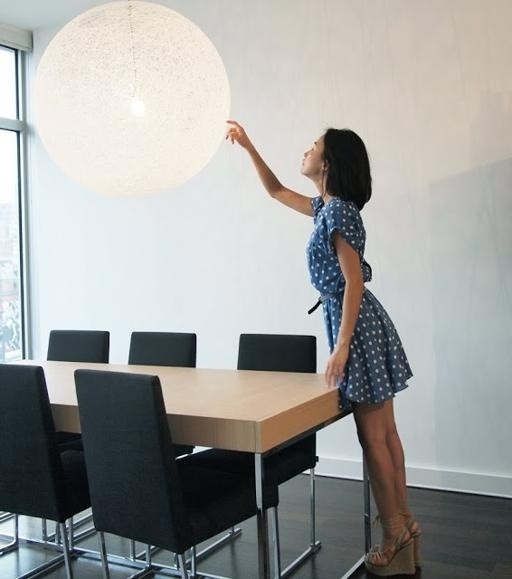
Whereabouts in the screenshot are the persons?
[223,118,422,575]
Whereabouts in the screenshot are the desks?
[0,358,373,579]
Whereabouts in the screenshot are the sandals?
[362,513,417,576]
[370,513,425,568]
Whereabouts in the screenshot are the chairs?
[128,331,201,458]
[173,333,324,578]
[1,363,92,579]
[73,366,281,579]
[45,330,110,364]
